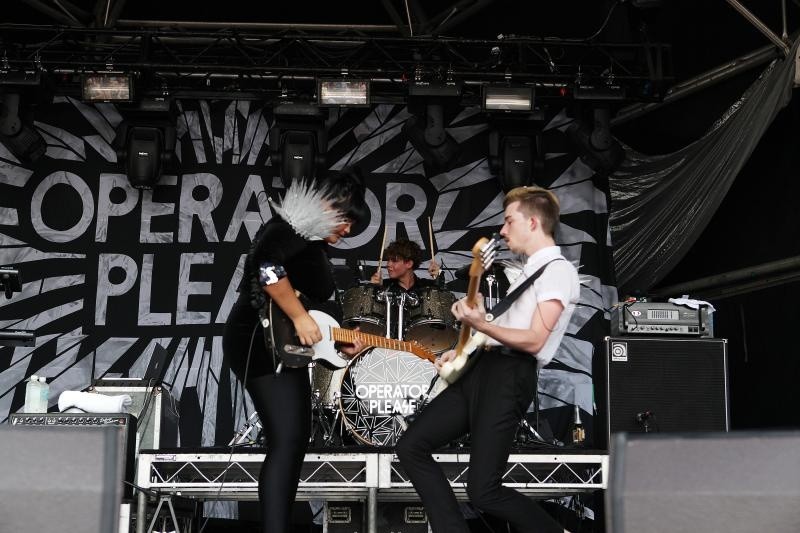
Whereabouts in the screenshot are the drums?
[338,345,448,451]
[404,290,457,355]
[340,285,386,337]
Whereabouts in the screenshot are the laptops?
[102,341,168,380]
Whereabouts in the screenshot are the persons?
[222,170,369,533]
[395,186,581,533]
[370,240,446,291]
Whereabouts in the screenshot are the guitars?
[262,288,435,372]
[440,236,498,384]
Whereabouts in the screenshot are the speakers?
[594,336,730,451]
[0,412,137,533]
[610,430,800,531]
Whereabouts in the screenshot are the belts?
[483,346,537,364]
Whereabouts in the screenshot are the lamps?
[274,90,323,117]
[480,79,535,114]
[317,75,371,106]
[82,64,136,105]
[408,67,462,96]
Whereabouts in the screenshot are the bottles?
[38,376,49,413]
[24,375,41,414]
[572,405,586,447]
